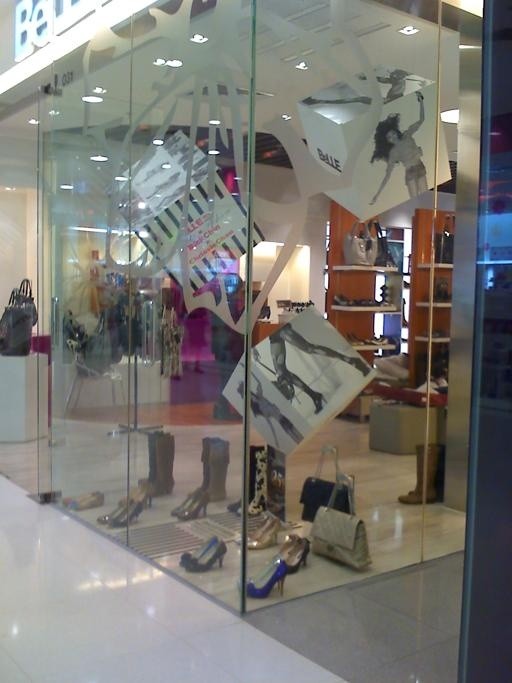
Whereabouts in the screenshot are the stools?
[367,398,436,456]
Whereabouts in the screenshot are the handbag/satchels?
[309,505,371,569]
[1,279,39,356]
[343,235,389,267]
[300,476,351,521]
[75,304,137,375]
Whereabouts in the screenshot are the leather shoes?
[213,405,240,420]
[345,331,389,346]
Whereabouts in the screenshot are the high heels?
[170,488,208,520]
[97,482,151,527]
[345,357,370,376]
[179,536,227,572]
[247,533,309,598]
[234,516,280,549]
[312,391,327,414]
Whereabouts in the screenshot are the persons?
[304,68,410,106]
[236,362,305,448]
[270,321,369,414]
[156,271,258,420]
[367,89,428,207]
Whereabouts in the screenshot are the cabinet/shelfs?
[328,200,454,424]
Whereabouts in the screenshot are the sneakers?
[417,376,448,393]
[64,490,106,511]
[334,294,380,306]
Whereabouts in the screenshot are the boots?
[398,443,445,503]
[187,437,229,500]
[227,446,269,518]
[138,430,175,496]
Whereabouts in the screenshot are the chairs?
[64,339,127,416]
